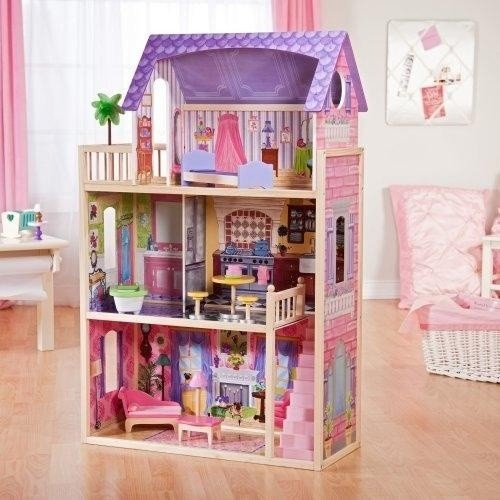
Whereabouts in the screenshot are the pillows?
[388,182,489,311]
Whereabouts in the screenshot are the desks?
[479,235,500,300]
[0,230,69,353]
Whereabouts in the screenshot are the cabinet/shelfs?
[77,30,368,471]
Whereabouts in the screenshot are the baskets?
[417,294,500,383]
